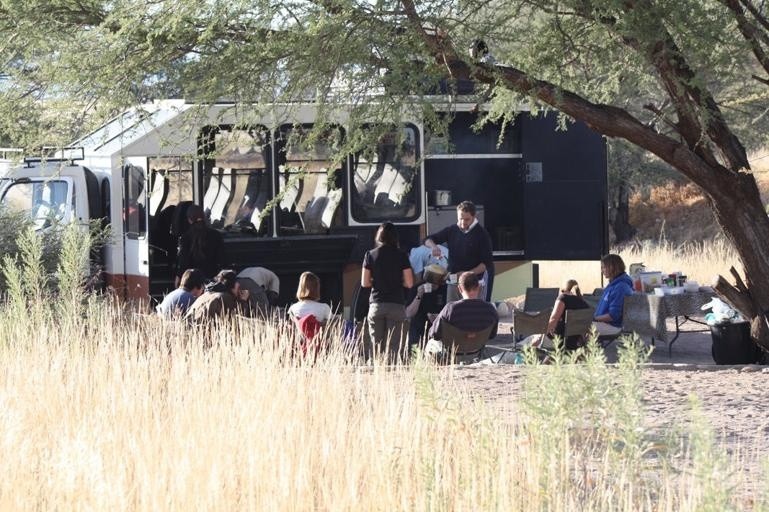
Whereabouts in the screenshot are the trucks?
[1,94,612,319]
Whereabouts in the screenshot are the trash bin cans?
[710,321,757,365]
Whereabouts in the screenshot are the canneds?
[668,274,677,287]
[679,275,687,286]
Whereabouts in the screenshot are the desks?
[653,286,715,359]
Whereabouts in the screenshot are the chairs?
[299,287,663,363]
[132,145,419,238]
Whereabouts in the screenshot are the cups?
[449,273,458,284]
[423,283,432,293]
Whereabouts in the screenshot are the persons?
[547,253,636,352]
[237,267,281,321]
[175,204,225,290]
[287,271,334,345]
[182,269,250,332]
[123,198,145,233]
[427,270,500,363]
[360,220,414,365]
[404,281,449,357]
[156,268,206,319]
[423,199,492,306]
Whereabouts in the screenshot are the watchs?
[415,295,420,300]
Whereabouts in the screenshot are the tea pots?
[422,252,452,286]
